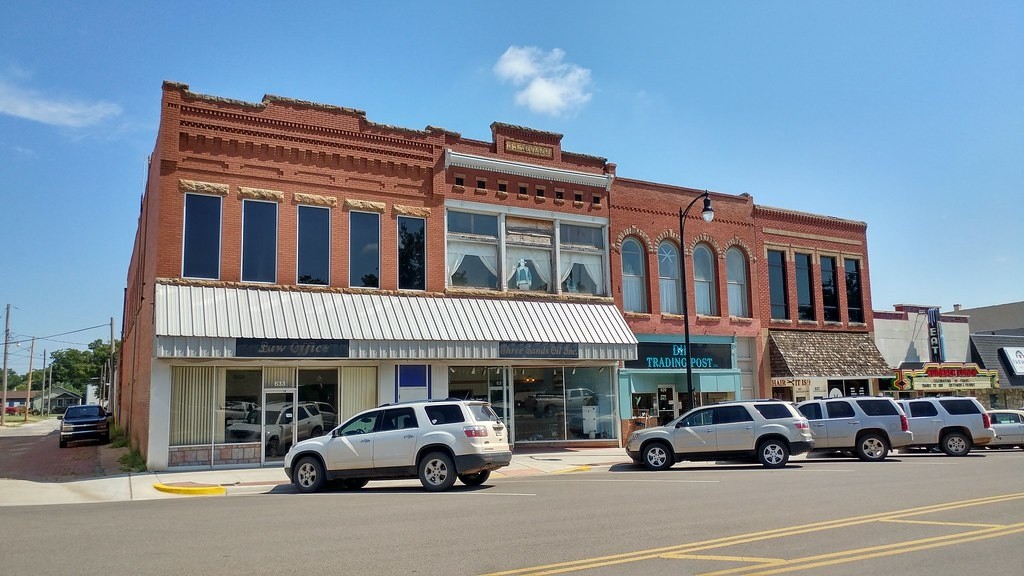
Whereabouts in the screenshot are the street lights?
[526,377,535,392]
[679,190,715,429]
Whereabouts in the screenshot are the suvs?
[624,398,815,469]
[226,400,325,455]
[492,400,510,431]
[794,395,914,461]
[284,397,512,494]
[894,396,997,458]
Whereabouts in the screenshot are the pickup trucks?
[301,400,338,429]
[527,387,605,419]
[212,400,260,426]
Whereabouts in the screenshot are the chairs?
[403,417,413,428]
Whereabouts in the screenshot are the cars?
[0,404,20,416]
[57,404,113,448]
[928,409,1024,453]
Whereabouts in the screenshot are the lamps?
[447,366,606,375]
